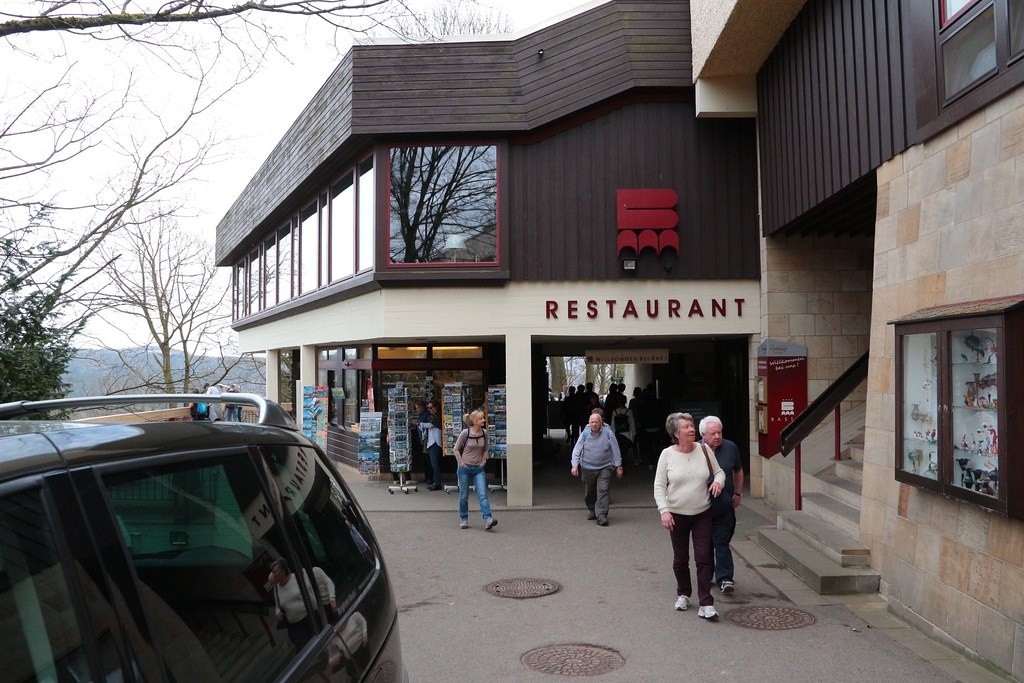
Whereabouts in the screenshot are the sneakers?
[721,580,734,593]
[697,606,719,618]
[674,595,690,610]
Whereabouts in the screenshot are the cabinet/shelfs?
[951,363,998,459]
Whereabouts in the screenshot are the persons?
[268,559,337,651]
[387,399,444,491]
[571,407,623,526]
[556,382,662,471]
[326,612,371,679]
[698,415,744,593]
[452,410,498,530]
[225,385,242,422]
[190,382,222,421]
[654,412,726,619]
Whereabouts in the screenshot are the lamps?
[170,531,188,544]
[443,234,471,261]
[622,258,637,271]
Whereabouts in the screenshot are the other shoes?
[427,479,433,484]
[587,512,595,520]
[394,480,400,484]
[427,483,440,491]
[597,513,608,525]
[406,479,417,485]
[485,518,498,530]
[634,458,640,466]
[461,521,468,529]
[418,479,426,483]
[566,436,571,443]
[639,457,643,462]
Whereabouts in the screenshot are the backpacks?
[197,402,207,414]
[614,409,628,432]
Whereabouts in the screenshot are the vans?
[0,392,410,681]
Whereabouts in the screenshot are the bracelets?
[736,493,742,496]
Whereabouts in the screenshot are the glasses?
[426,407,433,410]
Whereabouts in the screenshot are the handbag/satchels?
[699,443,733,517]
[276,613,287,629]
[332,654,345,674]
